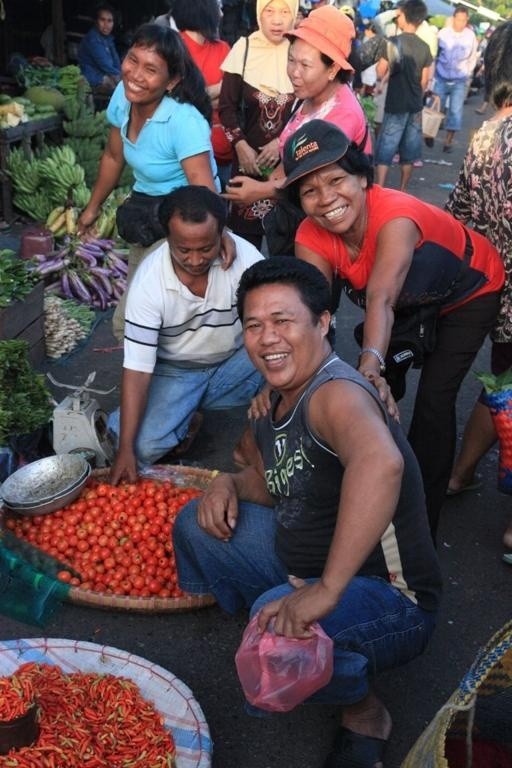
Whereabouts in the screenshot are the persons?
[444,17,512,565]
[174,257,443,768]
[76,1,476,262]
[282,120,505,552]
[108,188,267,486]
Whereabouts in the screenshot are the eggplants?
[31,238,130,310]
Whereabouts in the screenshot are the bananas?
[1,64,135,248]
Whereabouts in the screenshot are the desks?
[1,112,64,220]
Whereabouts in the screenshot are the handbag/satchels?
[422,96,444,137]
[116,195,168,247]
[354,302,437,366]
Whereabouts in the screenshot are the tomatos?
[6,475,205,596]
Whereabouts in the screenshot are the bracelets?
[358,348,389,374]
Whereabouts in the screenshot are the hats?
[282,119,350,188]
[282,6,356,70]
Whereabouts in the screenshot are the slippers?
[326,716,393,768]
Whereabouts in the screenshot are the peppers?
[0,661,178,767]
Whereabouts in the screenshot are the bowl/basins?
[0,452,93,518]
